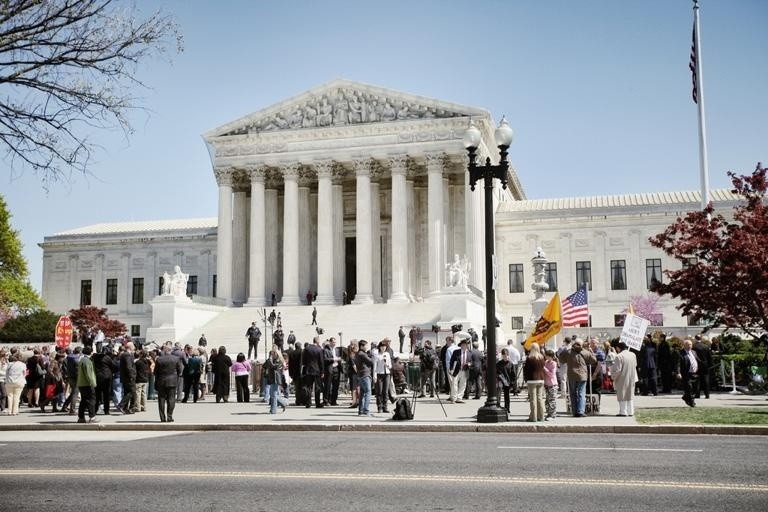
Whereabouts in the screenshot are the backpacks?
[393,399,413,420]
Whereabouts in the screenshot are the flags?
[688,12,699,102]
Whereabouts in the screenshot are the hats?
[458,340,470,346]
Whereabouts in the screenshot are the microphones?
[384,354,387,362]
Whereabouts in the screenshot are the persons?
[446,257,470,289]
[161,267,186,297]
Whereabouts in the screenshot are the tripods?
[410,358,448,418]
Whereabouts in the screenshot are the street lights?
[462,113,515,423]
[530,246,550,300]
